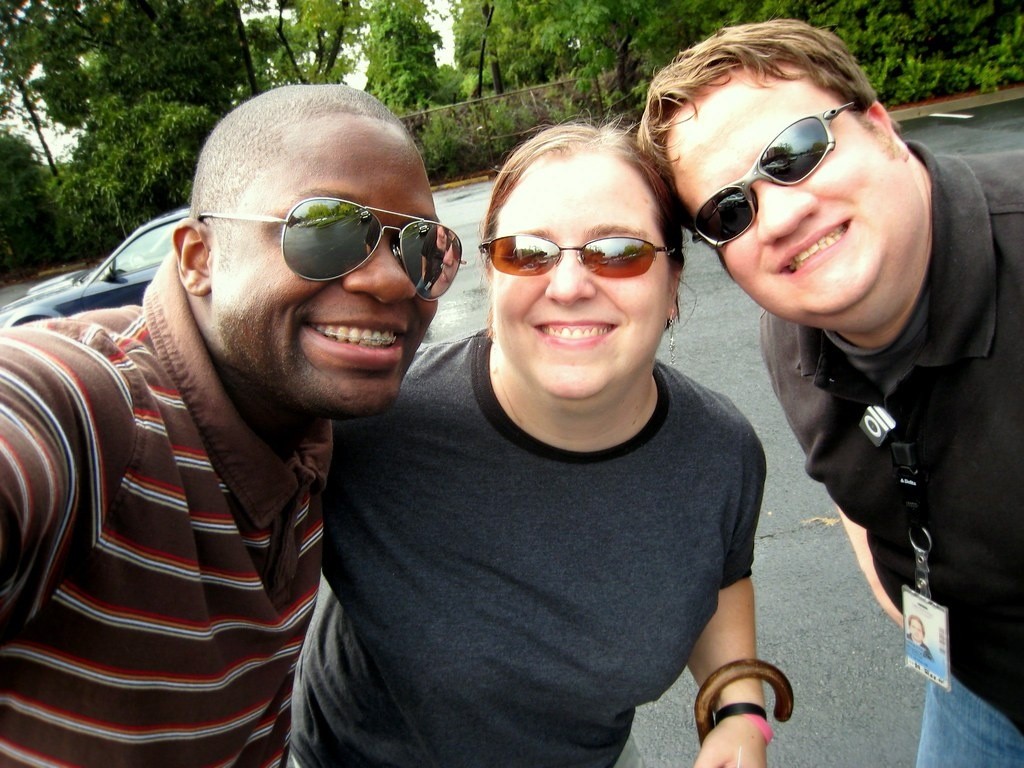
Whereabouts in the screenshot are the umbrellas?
[694,659,795,747]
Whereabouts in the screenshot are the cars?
[1,201,192,330]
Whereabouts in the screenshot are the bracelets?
[714,702,772,746]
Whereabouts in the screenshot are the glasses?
[478,235,678,279]
[691,102,852,248]
[198,197,467,303]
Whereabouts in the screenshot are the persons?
[904,614,934,660]
[286,126,774,768]
[0,83,465,768]
[637,19,1024,768]
